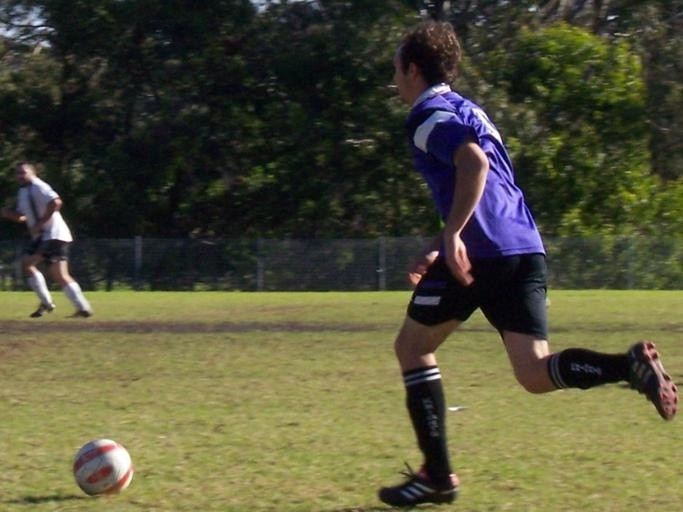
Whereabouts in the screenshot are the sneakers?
[376,460,462,508]
[27,301,55,319]
[624,337,680,423]
[62,308,94,320]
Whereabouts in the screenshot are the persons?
[377,21,678,507]
[0,161,95,316]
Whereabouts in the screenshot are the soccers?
[73,440,134,495]
